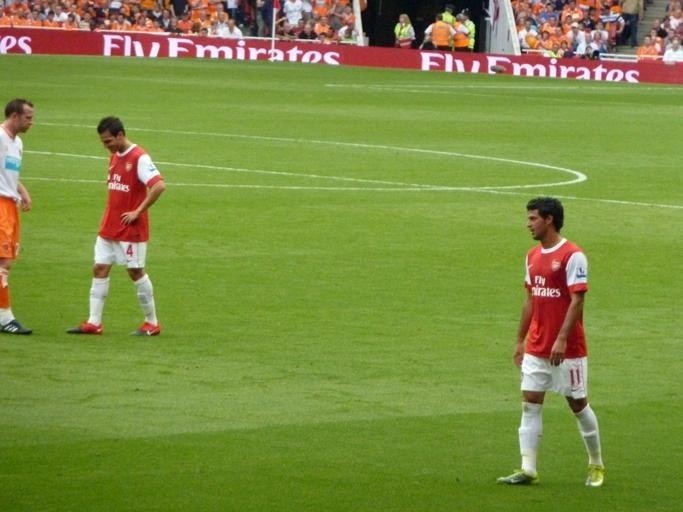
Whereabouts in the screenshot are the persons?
[395,14,416,49]
[0,98,34,335]
[1,0,367,43]
[510,1,643,62]
[66,116,167,336]
[420,4,476,53]
[637,1,681,66]
[495,196,605,487]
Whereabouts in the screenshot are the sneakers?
[66,321,103,334]
[499,470,539,485]
[0,319,32,334]
[130,321,160,335]
[585,464,604,486]
